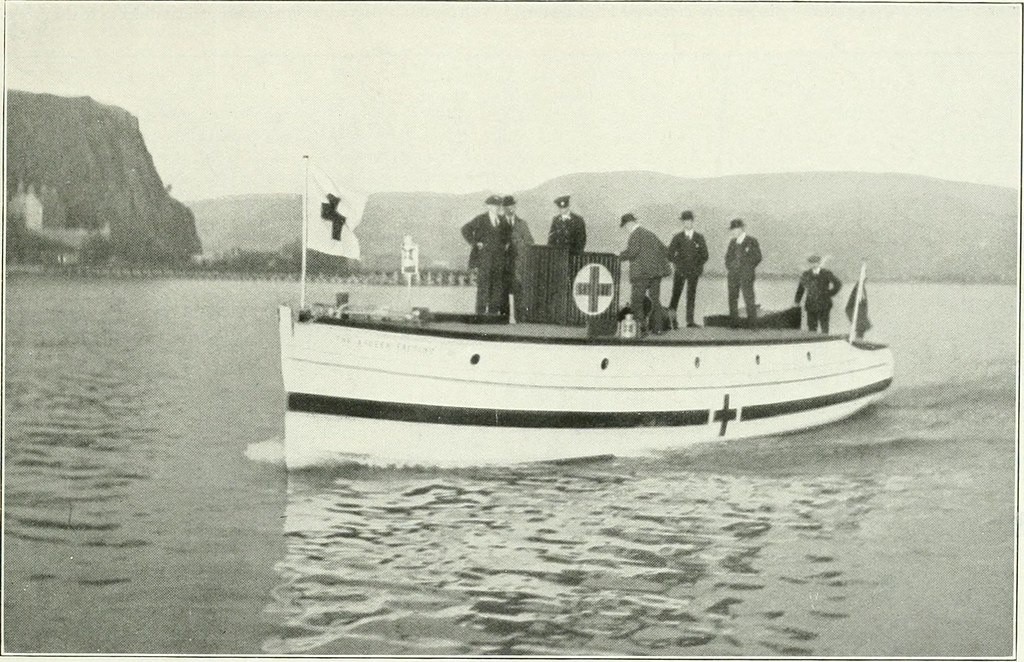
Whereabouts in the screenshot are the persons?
[544,196,587,251]
[666,211,708,331]
[461,194,514,314]
[725,217,763,329]
[612,213,671,338]
[499,196,535,322]
[792,256,842,334]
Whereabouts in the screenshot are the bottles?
[621,314,636,337]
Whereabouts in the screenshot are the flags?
[845,277,873,339]
[306,161,366,261]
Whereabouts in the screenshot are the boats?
[276,296,898,472]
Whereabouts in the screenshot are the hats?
[729,219,744,229]
[679,211,693,220]
[619,214,636,227]
[501,195,516,206]
[484,196,502,205]
[554,195,570,208]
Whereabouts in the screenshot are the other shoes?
[687,323,699,328]
